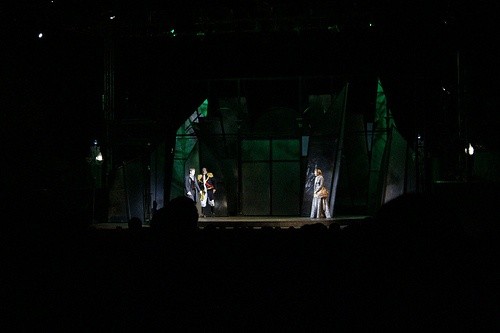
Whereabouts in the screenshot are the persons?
[0,193,499,333]
[309,168,332,220]
[184,167,202,207]
[197,167,216,219]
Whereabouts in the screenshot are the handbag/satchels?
[317,189,329,198]
